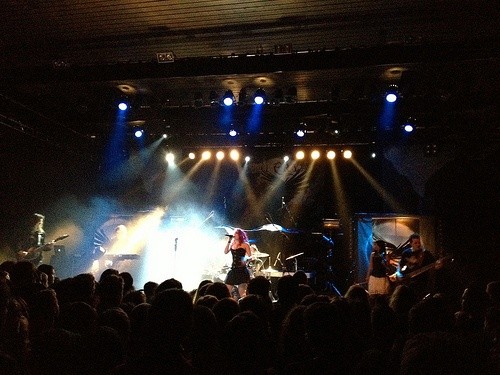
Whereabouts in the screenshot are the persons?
[248,244,265,271]
[0,256,500,375]
[14,212,48,266]
[222,228,252,297]
[365,239,393,295]
[399,232,437,285]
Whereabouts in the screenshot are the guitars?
[22,234,69,260]
[389,254,455,285]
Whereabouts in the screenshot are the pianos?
[72,252,141,269]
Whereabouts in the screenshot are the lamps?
[384,83,404,102]
[296,121,308,137]
[404,116,419,132]
[273,42,292,55]
[227,122,240,139]
[119,87,298,110]
[156,51,175,65]
[133,124,145,139]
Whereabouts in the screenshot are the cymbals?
[261,268,278,273]
[253,253,270,258]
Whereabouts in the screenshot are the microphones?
[225,235,235,237]
